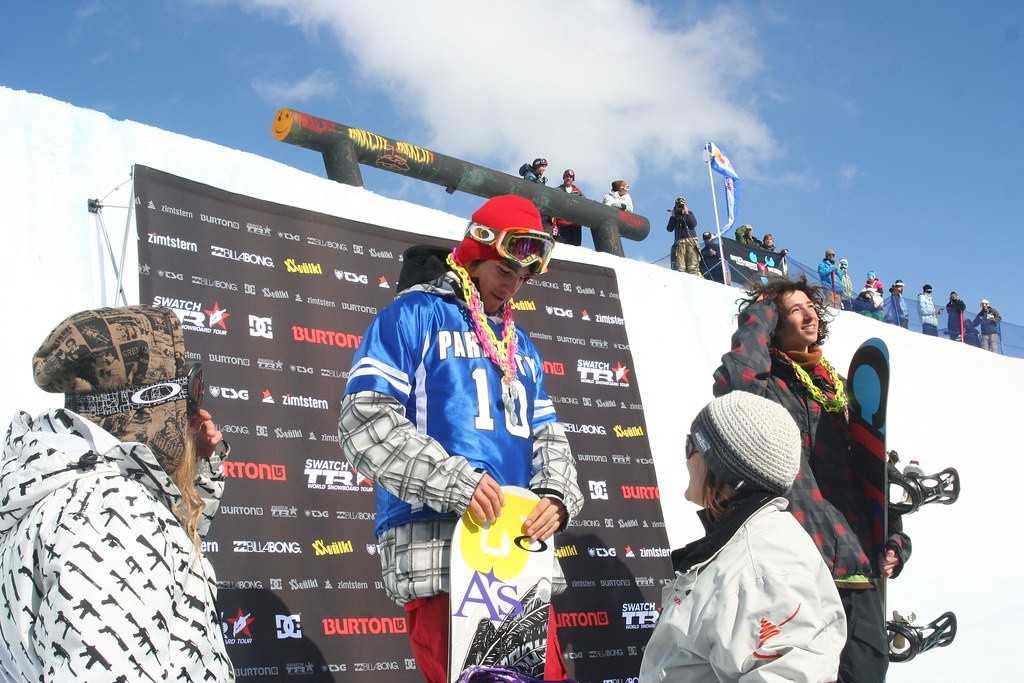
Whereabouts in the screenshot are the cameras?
[676,203,684,210]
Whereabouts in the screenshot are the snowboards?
[448,484,554,683]
[847,336,890,630]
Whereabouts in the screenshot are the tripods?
[667,209,714,282]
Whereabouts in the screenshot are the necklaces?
[778,350,847,413]
[446,247,518,427]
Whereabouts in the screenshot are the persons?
[946,291,966,340]
[0,304,236,683]
[712,279,912,683]
[337,194,584,683]
[818,249,854,311]
[638,389,847,683]
[552,169,581,246]
[602,180,633,213]
[519,158,552,236]
[699,231,724,284]
[852,271,884,321]
[964,318,982,348]
[889,280,909,329]
[667,197,699,275]
[973,299,1002,353]
[918,284,942,337]
[735,224,788,254]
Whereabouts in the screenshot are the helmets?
[923,284,932,292]
[895,280,903,287]
[867,271,875,278]
[839,258,847,264]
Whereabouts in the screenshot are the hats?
[825,249,834,258]
[703,232,710,239]
[533,158,543,168]
[982,300,989,306]
[690,389,801,498]
[457,194,543,283]
[563,170,575,181]
[612,181,622,190]
[184,362,204,414]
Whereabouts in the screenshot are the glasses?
[624,185,629,190]
[541,159,548,166]
[685,435,700,458]
[980,303,987,306]
[841,264,847,268]
[495,227,556,275]
